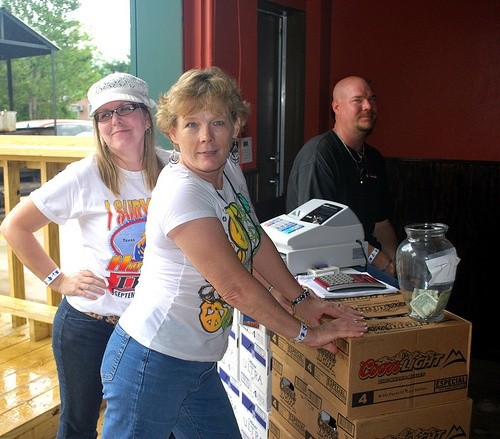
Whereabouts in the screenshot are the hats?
[87,73,156,117]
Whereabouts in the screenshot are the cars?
[0,118,93,209]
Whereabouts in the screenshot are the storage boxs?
[217,266,473,438]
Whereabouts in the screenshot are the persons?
[285,76,420,287]
[1,74,180,439]
[101,66,370,439]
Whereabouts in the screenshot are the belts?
[85,312,120,326]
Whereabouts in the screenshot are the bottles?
[396,223,458,323]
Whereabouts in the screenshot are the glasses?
[94,103,145,122]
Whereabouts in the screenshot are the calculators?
[312,271,388,293]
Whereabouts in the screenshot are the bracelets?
[291,288,309,306]
[292,322,308,343]
[381,260,392,272]
[368,248,379,262]
[43,267,62,286]
[268,286,273,293]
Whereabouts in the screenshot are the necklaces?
[334,129,376,183]
